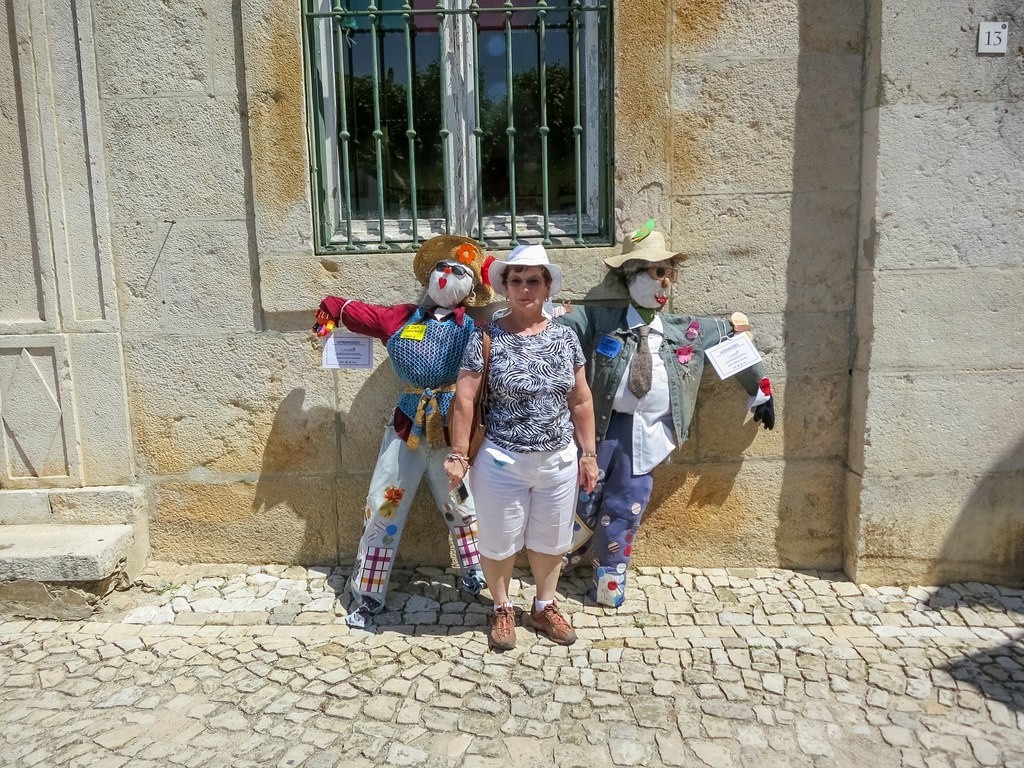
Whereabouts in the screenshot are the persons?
[313,235,489,628]
[552,230,776,609]
[444,245,599,650]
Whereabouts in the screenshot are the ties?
[628,327,651,399]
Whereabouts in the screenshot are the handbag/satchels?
[468,322,490,466]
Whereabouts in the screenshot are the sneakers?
[489,602,517,649]
[529,597,577,645]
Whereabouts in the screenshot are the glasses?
[635,267,679,282]
[430,262,474,279]
[506,274,546,287]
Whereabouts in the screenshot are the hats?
[413,235,495,307]
[602,219,688,270]
[488,244,561,297]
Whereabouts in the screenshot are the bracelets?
[581,453,597,459]
[446,453,470,467]
